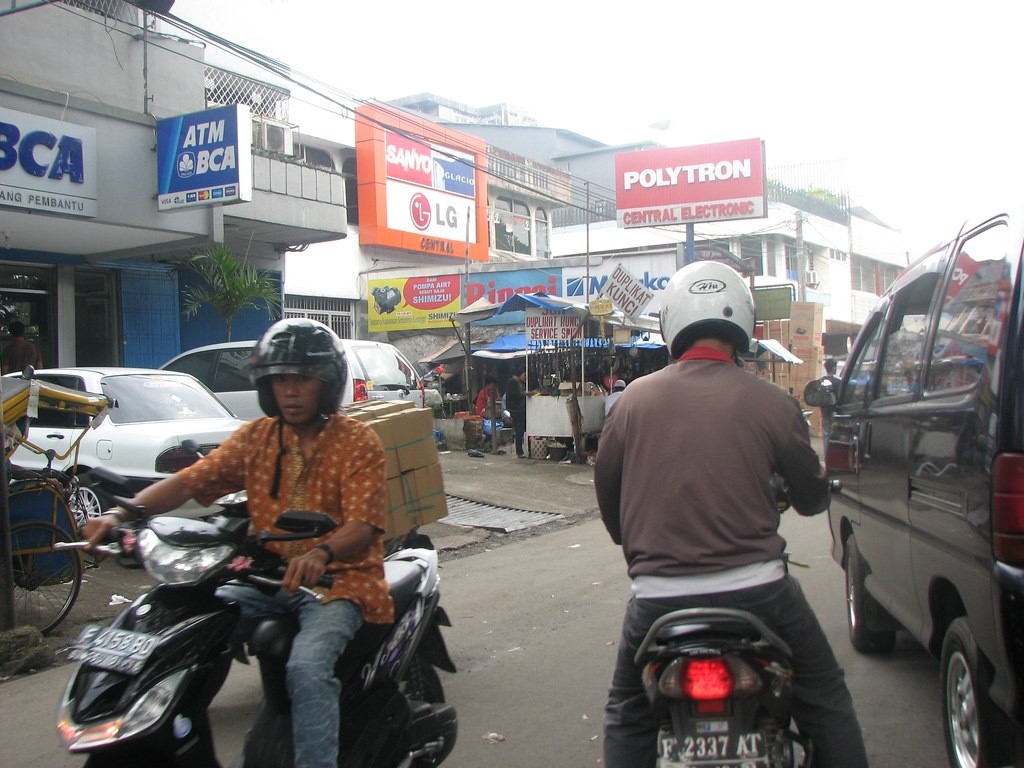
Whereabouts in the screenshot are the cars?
[2,366,253,556]
[157,338,425,426]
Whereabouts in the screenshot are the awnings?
[757,338,804,366]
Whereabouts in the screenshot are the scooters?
[633,476,843,768]
[180,440,435,666]
[53,496,459,768]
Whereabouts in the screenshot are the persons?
[605,379,626,418]
[475,378,506,455]
[501,365,536,459]
[595,261,868,768]
[83,317,395,768]
[603,368,618,392]
[819,357,841,460]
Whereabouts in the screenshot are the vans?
[802,208,1024,767]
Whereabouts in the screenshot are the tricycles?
[1,376,104,636]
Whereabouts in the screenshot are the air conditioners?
[805,271,819,289]
[253,116,294,156]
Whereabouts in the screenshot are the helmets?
[615,379,626,388]
[247,317,348,418]
[658,259,755,359]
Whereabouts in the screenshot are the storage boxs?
[742,299,827,439]
[338,398,450,545]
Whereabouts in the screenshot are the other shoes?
[518,454,526,458]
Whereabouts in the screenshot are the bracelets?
[315,544,334,565]
[103,510,124,522]
